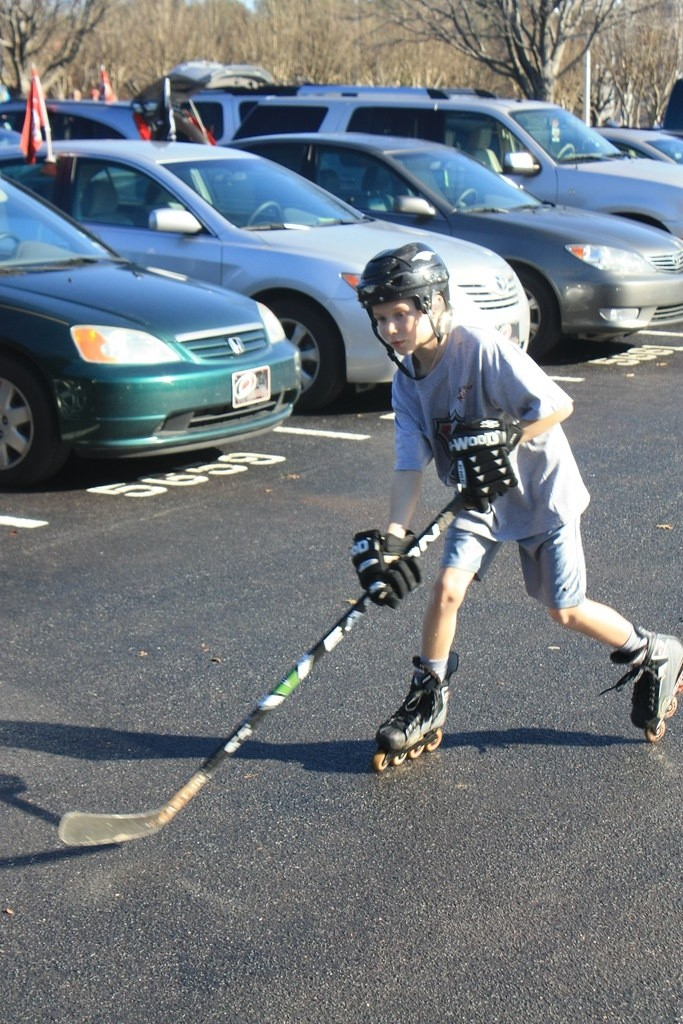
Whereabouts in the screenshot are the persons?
[356,242,683,771]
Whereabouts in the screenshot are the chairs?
[136,182,180,227]
[359,166,397,213]
[318,170,348,205]
[467,127,502,174]
[81,179,134,226]
[444,130,455,149]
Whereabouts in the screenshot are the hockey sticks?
[56,494,464,850]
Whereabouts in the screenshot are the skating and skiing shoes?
[598,625,683,742]
[373,653,457,771]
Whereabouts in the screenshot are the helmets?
[355,243,452,313]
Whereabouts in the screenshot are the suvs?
[217,83,683,242]
[131,61,277,104]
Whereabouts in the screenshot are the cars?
[0,172,304,492]
[591,127,683,164]
[215,132,683,362]
[0,140,531,415]
[0,98,210,146]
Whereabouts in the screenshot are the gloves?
[353,530,425,609]
[449,417,523,513]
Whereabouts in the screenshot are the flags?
[98,69,117,102]
[19,70,48,166]
